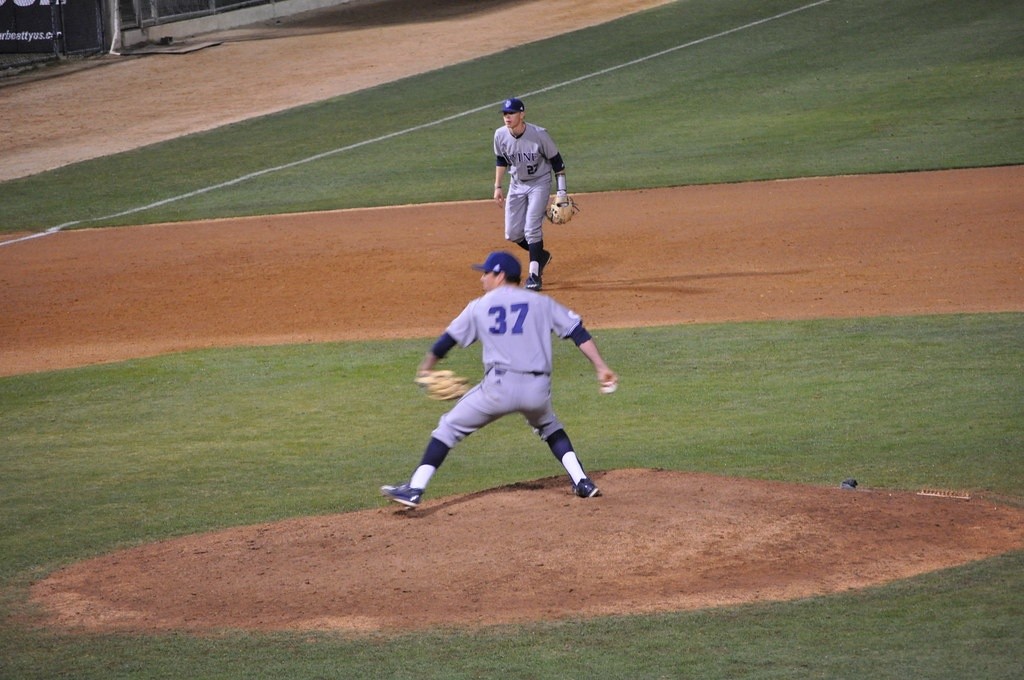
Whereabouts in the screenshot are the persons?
[490,97,575,293]
[379,250,622,509]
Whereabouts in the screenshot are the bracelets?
[494,186,501,189]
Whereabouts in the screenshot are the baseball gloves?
[547,195,580,224]
[414,369,472,401]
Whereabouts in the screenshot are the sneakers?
[540,249,552,276]
[572,478,600,498]
[524,273,543,291]
[379,482,425,508]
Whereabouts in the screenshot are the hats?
[498,98,525,113]
[468,250,522,279]
